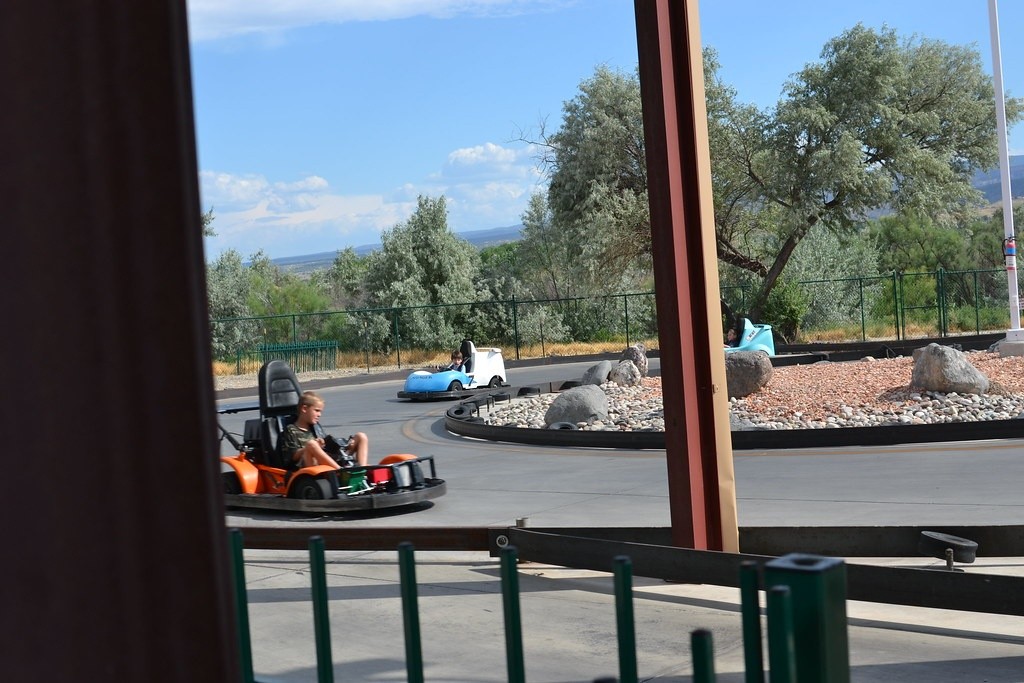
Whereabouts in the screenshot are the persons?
[722,328,740,349]
[278,392,367,480]
[440,349,466,374]
